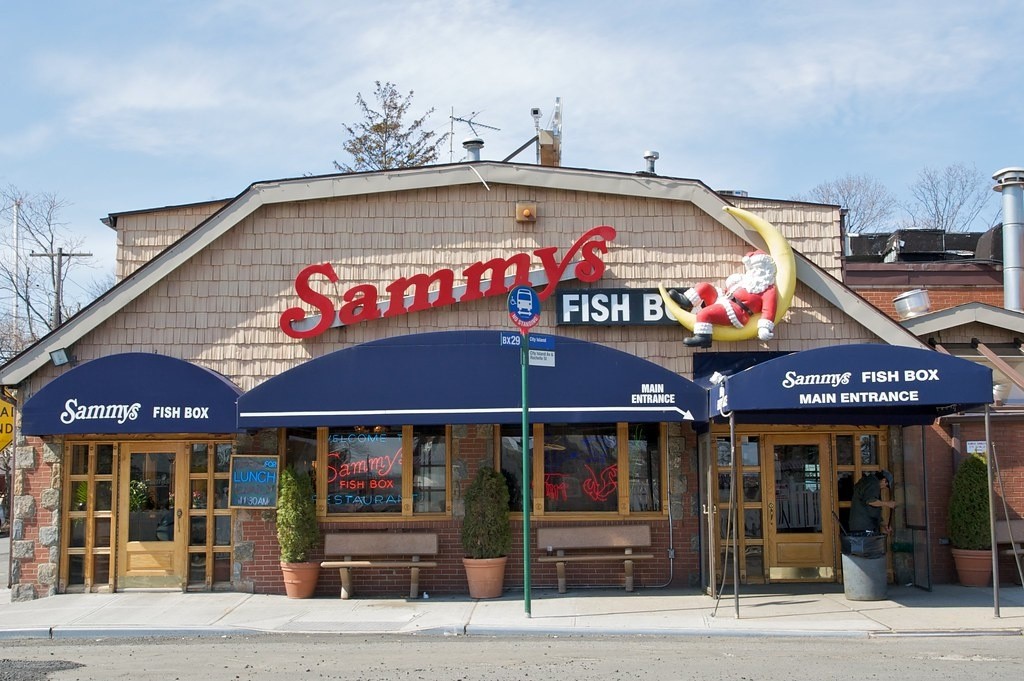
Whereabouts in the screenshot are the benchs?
[536,525,656,594]
[995,520,1024,586]
[319,532,439,599]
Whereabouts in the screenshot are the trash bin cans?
[842,531,888,601]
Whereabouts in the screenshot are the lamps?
[49,347,79,368]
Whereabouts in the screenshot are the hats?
[880,469,894,490]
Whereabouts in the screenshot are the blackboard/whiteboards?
[229,455,279,510]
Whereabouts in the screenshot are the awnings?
[21,351,248,434]
[706,341,1005,620]
[236,331,712,436]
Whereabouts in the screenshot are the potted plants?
[274,465,320,600]
[459,466,514,600]
[947,448,997,589]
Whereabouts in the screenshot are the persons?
[848,470,898,534]
[670,250,780,347]
